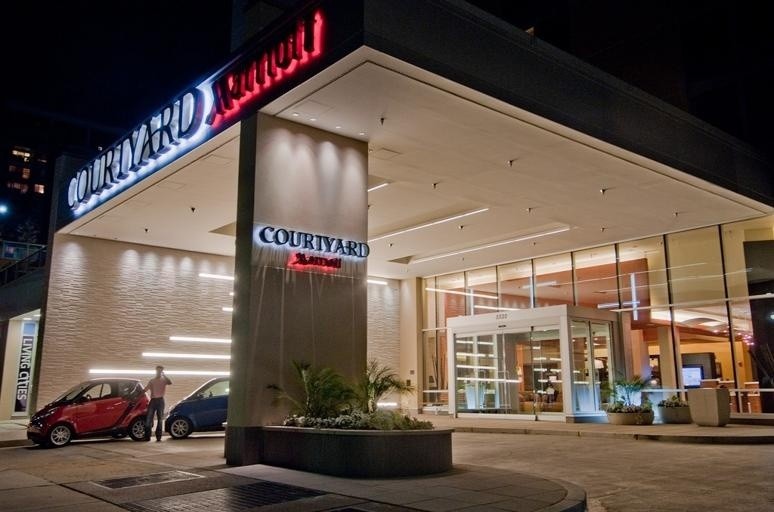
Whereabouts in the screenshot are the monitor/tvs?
[682,365,704,388]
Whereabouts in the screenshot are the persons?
[137,365,172,441]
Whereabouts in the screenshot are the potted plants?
[259,357,453,477]
[599,369,653,424]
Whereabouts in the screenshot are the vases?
[658,406,691,425]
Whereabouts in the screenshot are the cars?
[163,376,230,439]
[25,377,152,451]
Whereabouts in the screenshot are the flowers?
[657,394,688,407]
